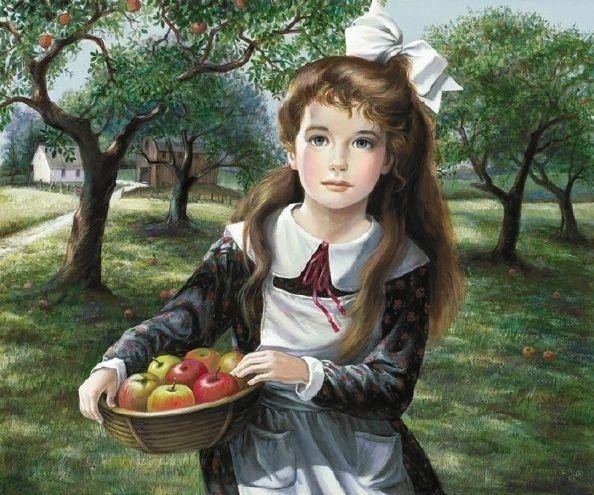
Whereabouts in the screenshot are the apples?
[0,0,556,413]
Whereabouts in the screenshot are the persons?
[75,0,462,494]
